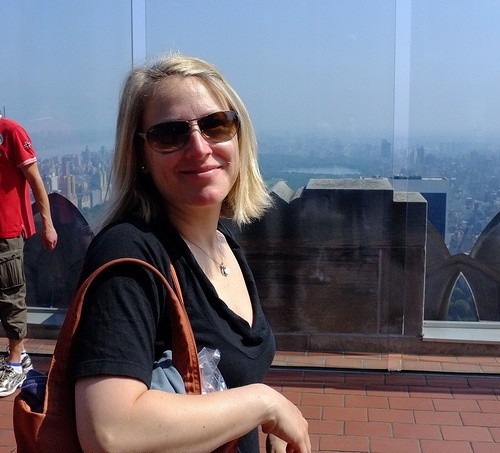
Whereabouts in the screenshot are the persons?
[63,49,313,452]
[0,114,58,397]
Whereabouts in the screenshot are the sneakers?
[0,345,34,397]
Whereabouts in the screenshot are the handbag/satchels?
[12,258,240,453]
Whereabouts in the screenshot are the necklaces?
[179,229,231,278]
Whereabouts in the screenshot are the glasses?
[134,110,240,154]
